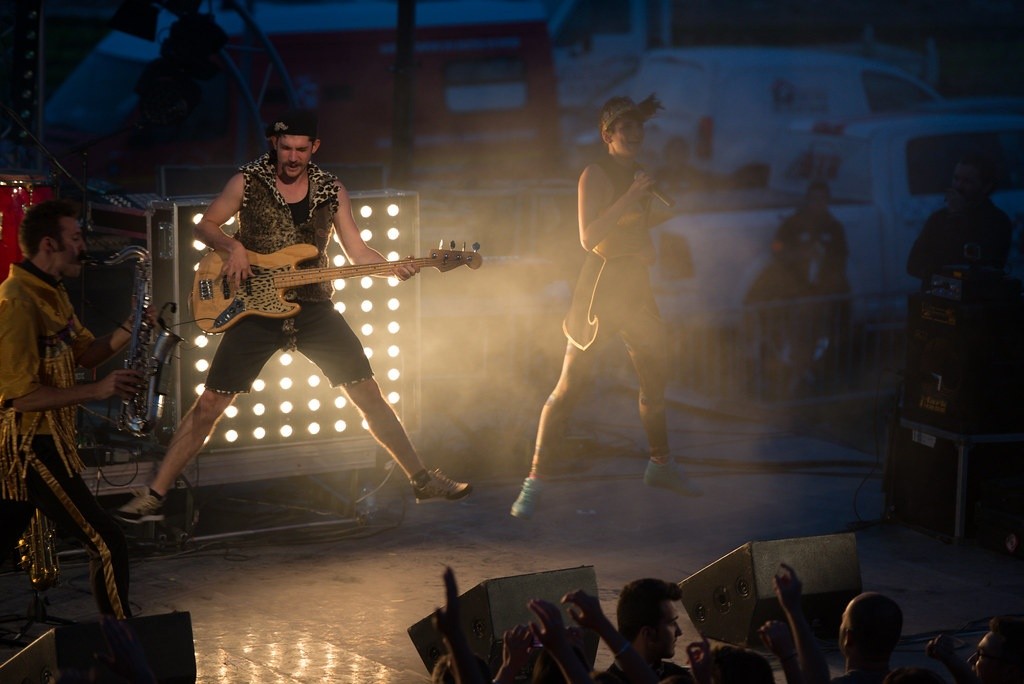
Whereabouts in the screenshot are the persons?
[0,199,130,620]
[509,97,709,516]
[432,162,1024,684]
[112,112,474,525]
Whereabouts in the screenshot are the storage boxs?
[892,415,1024,546]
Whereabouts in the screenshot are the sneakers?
[116,486,167,523]
[409,468,472,504]
[644,456,705,497]
[510,477,543,519]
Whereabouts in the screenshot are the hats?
[600,97,643,130]
[265,108,320,139]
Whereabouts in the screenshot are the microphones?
[633,171,672,207]
[170,303,176,313]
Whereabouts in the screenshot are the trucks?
[443,45,1022,342]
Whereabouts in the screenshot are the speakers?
[0,610,197,684]
[677,532,863,649]
[407,566,601,684]
